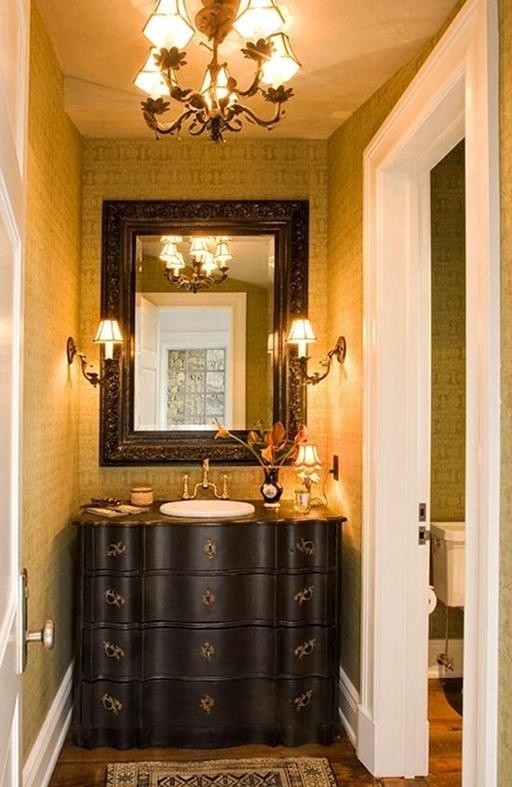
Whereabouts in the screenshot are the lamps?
[292,442,335,510]
[157,232,234,295]
[286,315,346,387]
[134,0,304,147]
[67,310,124,388]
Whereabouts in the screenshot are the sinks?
[160,500,255,519]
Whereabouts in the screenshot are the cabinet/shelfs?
[71,526,343,751]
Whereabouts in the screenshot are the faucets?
[203,459,210,489]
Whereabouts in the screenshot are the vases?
[259,468,285,507]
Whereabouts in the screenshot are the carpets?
[104,757,337,786]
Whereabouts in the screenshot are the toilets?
[431,520,466,608]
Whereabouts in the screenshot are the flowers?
[212,416,309,468]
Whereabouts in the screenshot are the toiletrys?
[130,486,155,506]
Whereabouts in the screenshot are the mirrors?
[98,198,310,468]
